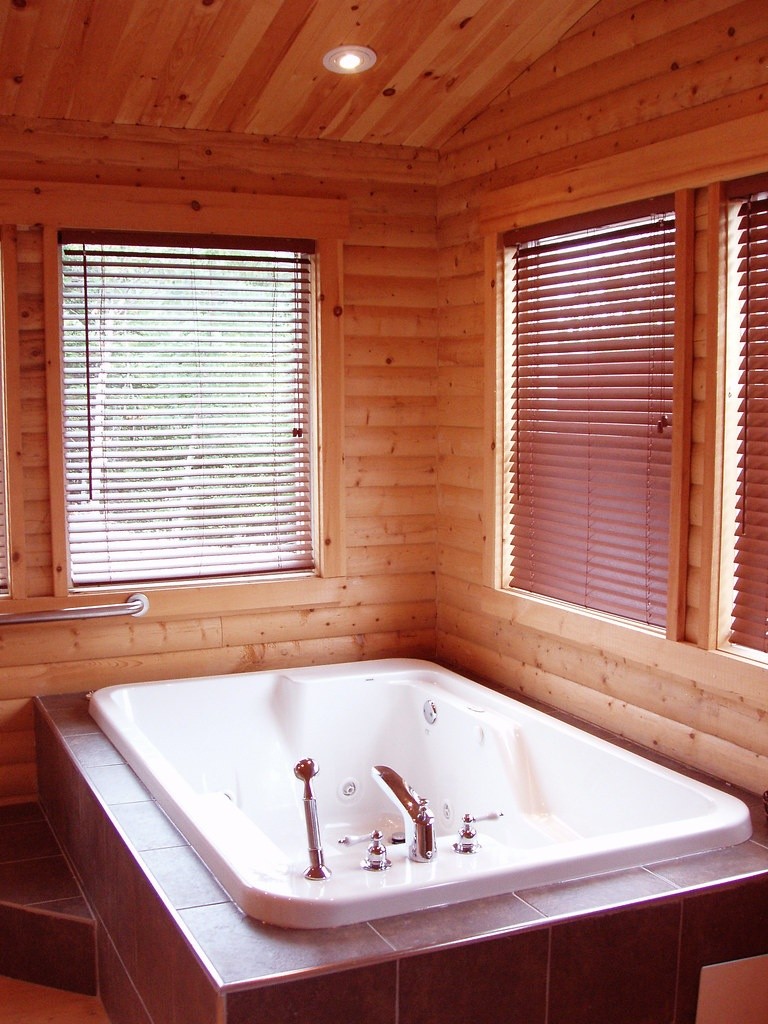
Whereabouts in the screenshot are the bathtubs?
[33,656,766,1024]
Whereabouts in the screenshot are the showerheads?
[293,755,334,880]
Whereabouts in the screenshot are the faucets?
[371,765,438,863]
